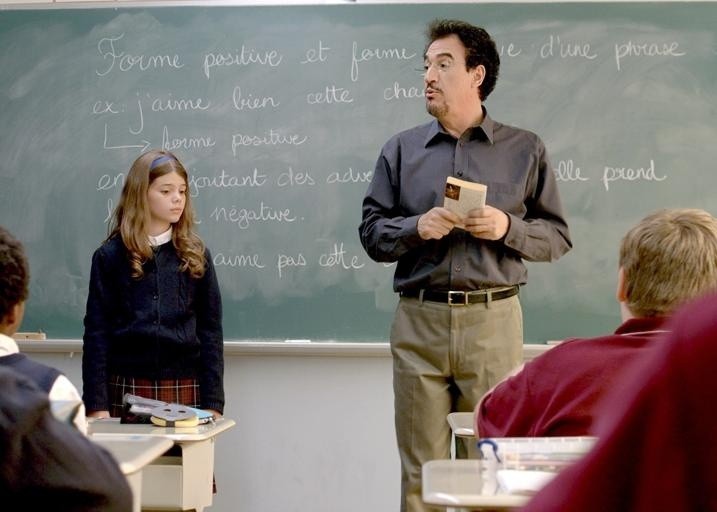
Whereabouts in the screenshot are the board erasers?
[12,332,46,340]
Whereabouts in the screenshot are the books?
[442,176,487,233]
[484,436,600,492]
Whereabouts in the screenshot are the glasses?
[415,59,466,73]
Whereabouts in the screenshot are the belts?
[398,287,521,306]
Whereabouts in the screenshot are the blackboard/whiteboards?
[0,0,717,356]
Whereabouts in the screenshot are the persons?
[0,226,91,439]
[520,281,717,510]
[81,148,226,497]
[358,20,573,511]
[470,208,717,432]
[0,365,135,512]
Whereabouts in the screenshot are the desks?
[83,419,238,512]
[418,411,607,512]
